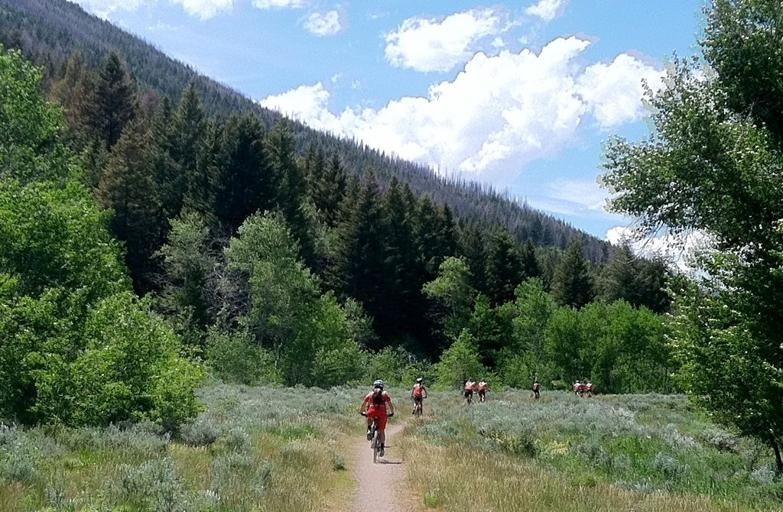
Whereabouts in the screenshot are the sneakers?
[380,449,384,456]
[367,431,371,441]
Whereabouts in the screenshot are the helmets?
[417,378,422,382]
[374,380,384,387]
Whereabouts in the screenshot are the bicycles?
[464,388,593,405]
[359,411,393,462]
[409,395,427,419]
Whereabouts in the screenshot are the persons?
[585,381,592,398]
[532,380,540,400]
[578,381,586,398]
[464,377,475,404]
[409,378,428,414]
[477,378,487,403]
[573,379,581,394]
[358,379,396,456]
